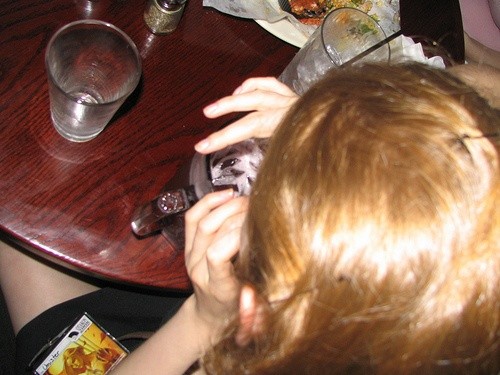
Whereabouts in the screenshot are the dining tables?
[0,0,465,292]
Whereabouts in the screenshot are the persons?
[1,59,500,375]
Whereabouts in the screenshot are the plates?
[254,18,317,48]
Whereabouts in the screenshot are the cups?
[45,20,142,143]
[131,137,268,236]
[278,7,391,97]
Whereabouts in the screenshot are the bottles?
[143,0,187,35]
